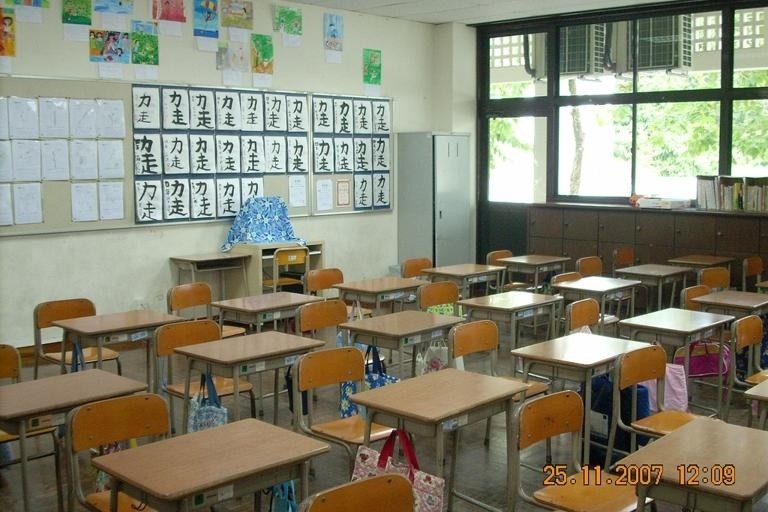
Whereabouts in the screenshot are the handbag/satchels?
[671,338,734,380]
[337,300,378,358]
[350,429,445,512]
[91,442,125,494]
[338,342,401,424]
[185,373,228,435]
[630,341,689,417]
[415,337,468,432]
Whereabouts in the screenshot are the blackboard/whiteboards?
[0,77,395,235]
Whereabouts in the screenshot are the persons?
[90,32,130,57]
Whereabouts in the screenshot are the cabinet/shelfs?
[397,131,475,266]
[529,201,768,309]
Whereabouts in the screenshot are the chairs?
[292,346,414,482]
[299,474,416,512]
[154,320,257,434]
[743,257,763,293]
[416,281,489,355]
[67,394,217,512]
[565,298,617,378]
[697,267,730,293]
[612,246,658,313]
[0,343,64,512]
[448,319,552,464]
[723,315,767,427]
[551,272,619,337]
[167,282,250,383]
[575,256,633,328]
[680,284,711,311]
[508,390,656,512]
[305,268,373,335]
[262,247,310,334]
[401,258,464,317]
[486,250,543,296]
[296,300,386,376]
[603,341,697,474]
[33,298,122,380]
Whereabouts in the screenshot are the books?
[698,179,718,212]
[636,197,690,209]
[746,185,767,212]
[720,182,742,211]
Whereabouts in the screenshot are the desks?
[52,309,183,394]
[511,331,654,467]
[690,290,768,405]
[212,290,324,340]
[668,254,736,290]
[224,237,326,330]
[553,275,641,340]
[497,254,571,294]
[421,264,506,316]
[615,264,692,342]
[457,290,563,377]
[0,368,148,512]
[332,279,425,316]
[91,418,331,512]
[743,379,768,431]
[170,252,253,331]
[615,417,767,512]
[349,368,529,512]
[756,281,768,294]
[339,310,466,379]
[174,331,326,435]
[617,307,736,417]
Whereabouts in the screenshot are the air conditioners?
[609,14,693,80]
[532,23,605,83]
